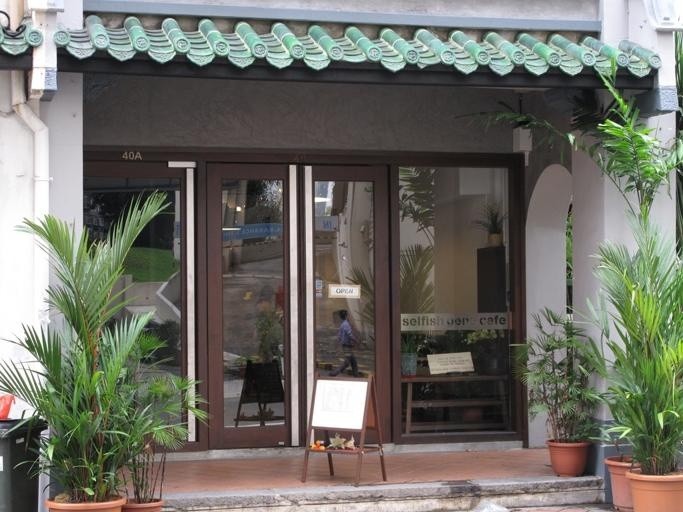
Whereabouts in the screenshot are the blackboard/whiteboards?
[250,359,283,402]
[307,376,373,432]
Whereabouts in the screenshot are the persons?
[325,310,361,378]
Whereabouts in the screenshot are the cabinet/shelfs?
[401,375,512,433]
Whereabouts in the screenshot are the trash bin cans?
[0,420,48,512]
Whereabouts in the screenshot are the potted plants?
[235,355,260,376]
[123,331,215,512]
[401,332,418,376]
[0,187,194,512]
[455,53,683,512]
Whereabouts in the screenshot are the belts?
[342,344,352,347]
